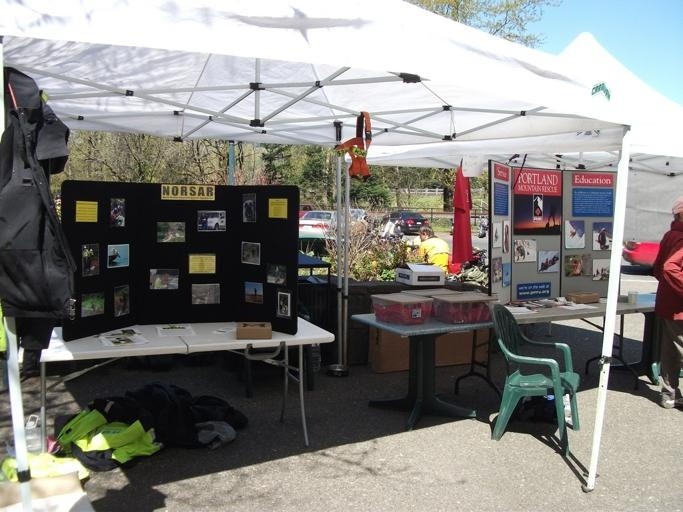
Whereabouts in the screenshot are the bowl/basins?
[326,364,349,378]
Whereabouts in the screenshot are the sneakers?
[660,387,683,408]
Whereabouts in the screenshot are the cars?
[206,211,226,230]
[299,209,429,238]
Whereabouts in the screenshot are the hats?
[672,195,683,215]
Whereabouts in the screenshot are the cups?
[628,290,638,306]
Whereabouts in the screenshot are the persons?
[596,227,606,250]
[651,195,683,411]
[416,225,450,274]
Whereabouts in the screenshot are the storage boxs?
[370,260,499,325]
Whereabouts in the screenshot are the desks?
[455,297,657,402]
[351,313,494,431]
[40,316,335,455]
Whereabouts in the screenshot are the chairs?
[491,303,581,457]
